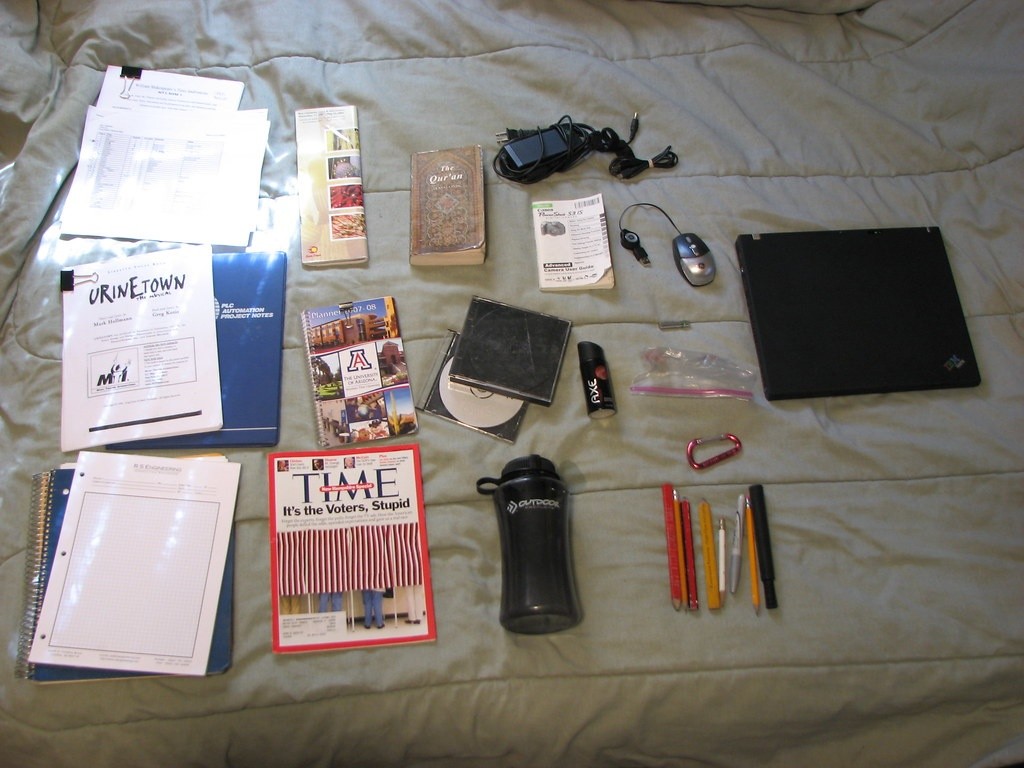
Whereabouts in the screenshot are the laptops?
[735,226,981,401]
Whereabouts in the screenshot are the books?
[17,454,243,686]
[532,194,613,293]
[297,103,368,268]
[104,249,288,449]
[409,143,487,267]
[268,297,438,656]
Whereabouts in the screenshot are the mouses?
[672,232,716,287]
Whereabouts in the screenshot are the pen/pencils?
[661,482,778,618]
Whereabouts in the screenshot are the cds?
[439,355,524,427]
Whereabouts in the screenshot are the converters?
[502,127,583,170]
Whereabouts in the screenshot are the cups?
[476,455,584,635]
